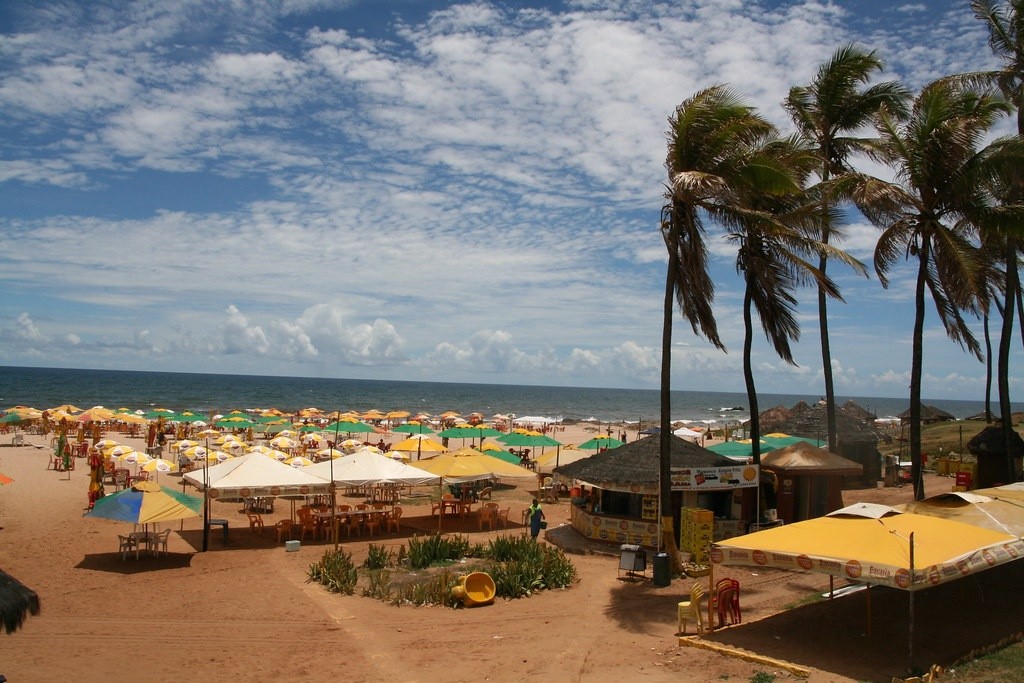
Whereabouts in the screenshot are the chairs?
[4,419,566,562]
[676,577,742,639]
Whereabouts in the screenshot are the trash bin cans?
[651,550,672,587]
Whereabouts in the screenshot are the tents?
[0,401,740,560]
[710,502,1023,673]
[704,438,775,463]
[890,481,1024,537]
[741,390,1002,450]
